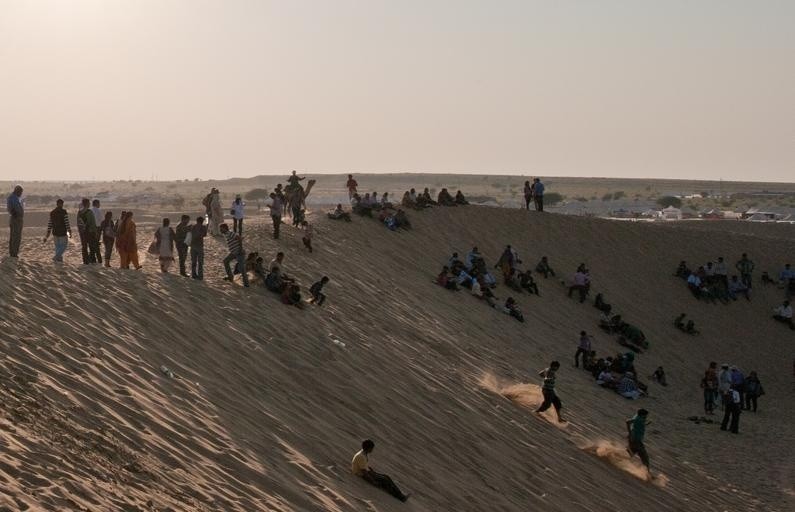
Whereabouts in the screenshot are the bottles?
[159,365,174,378]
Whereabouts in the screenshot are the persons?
[432,246,649,422]
[333,203,352,223]
[43,197,143,270]
[219,224,307,310]
[6,185,26,259]
[524,178,545,212]
[266,170,307,239]
[154,215,207,280]
[346,175,413,232]
[348,440,408,506]
[309,276,329,306]
[670,253,795,337]
[403,188,470,211]
[648,366,667,387]
[303,222,314,252]
[701,361,766,434]
[202,188,246,235]
[625,408,651,474]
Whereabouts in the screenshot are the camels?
[283,180,316,218]
